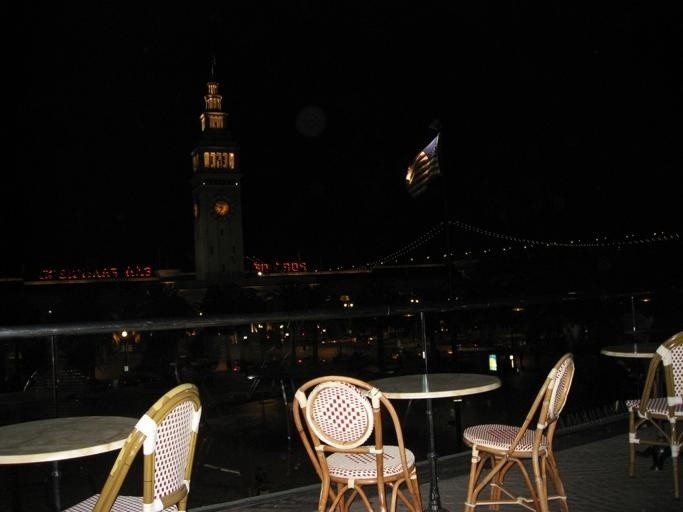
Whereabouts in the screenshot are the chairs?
[53,378,203,512]
[455,349,583,512]
[288,373,425,512]
[621,327,683,501]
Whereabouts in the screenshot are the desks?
[356,368,504,511]
[0,417,150,510]
[598,338,682,473]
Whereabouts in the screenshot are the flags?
[406,132,441,198]
